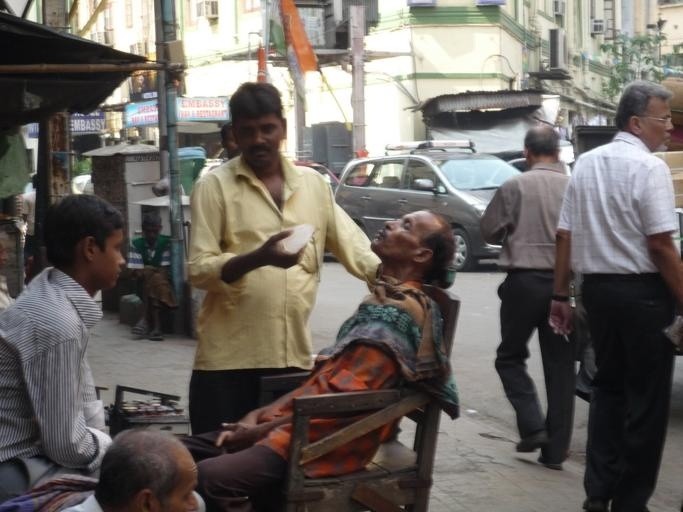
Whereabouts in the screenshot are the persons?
[184,81,384,436]
[21,174,36,283]
[0,217,20,307]
[61,427,198,512]
[0,193,127,511]
[176,211,457,510]
[546,78,680,510]
[476,120,573,471]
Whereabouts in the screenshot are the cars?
[197,157,229,186]
[332,140,523,272]
[291,160,341,198]
[69,172,94,196]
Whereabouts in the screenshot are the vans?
[178,146,206,197]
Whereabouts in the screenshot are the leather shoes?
[516,429,547,452]
[538,456,561,470]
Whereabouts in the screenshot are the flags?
[263,0,323,114]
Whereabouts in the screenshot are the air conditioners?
[204,1,217,18]
[547,27,569,73]
[594,19,603,33]
[130,41,145,56]
[88,32,104,43]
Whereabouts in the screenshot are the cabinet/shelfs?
[103,405,189,440]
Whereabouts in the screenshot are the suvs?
[571,124,682,379]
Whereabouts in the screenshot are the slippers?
[131,320,148,335]
[149,329,163,341]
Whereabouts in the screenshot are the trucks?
[427,116,575,171]
[22,137,39,195]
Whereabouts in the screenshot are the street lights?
[647,16,667,85]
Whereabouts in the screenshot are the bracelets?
[550,292,571,302]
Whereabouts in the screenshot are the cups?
[282,223,315,254]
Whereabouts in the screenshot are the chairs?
[379,174,400,188]
[256,285,459,512]
